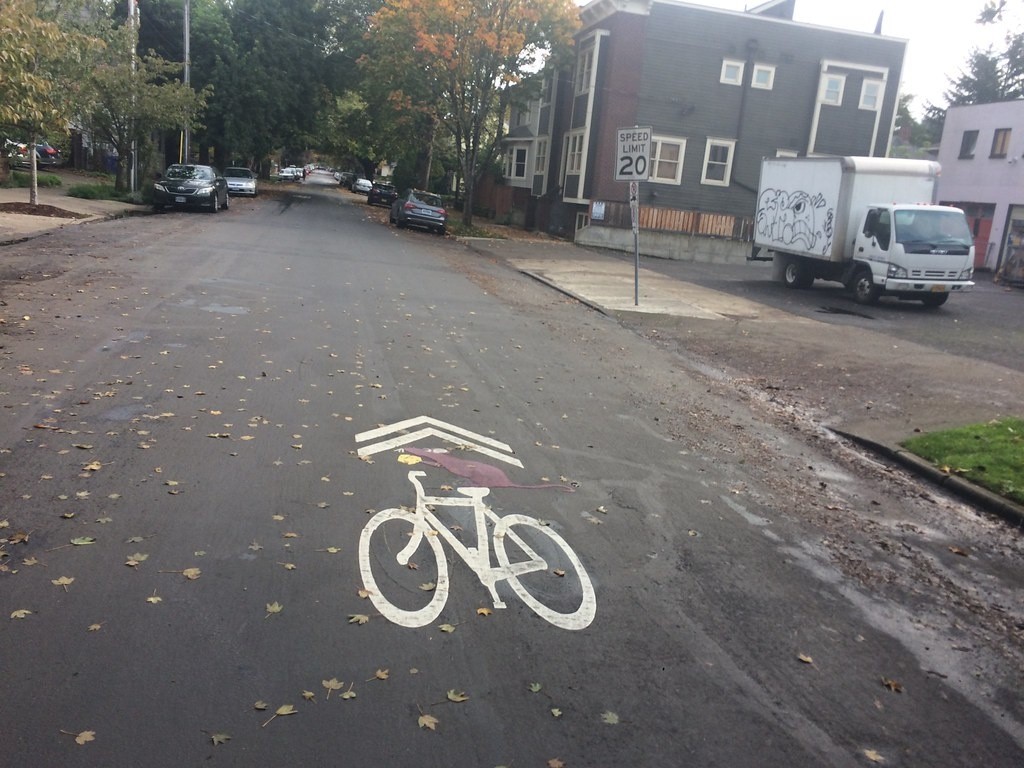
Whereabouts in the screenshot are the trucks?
[745,154,978,309]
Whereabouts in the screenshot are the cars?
[277,161,319,182]
[390,186,447,234]
[222,166,258,198]
[3,138,60,170]
[351,178,374,194]
[152,163,230,213]
[366,182,398,205]
[333,170,357,191]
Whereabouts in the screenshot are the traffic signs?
[614,125,653,182]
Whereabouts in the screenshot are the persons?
[303,169,307,180]
